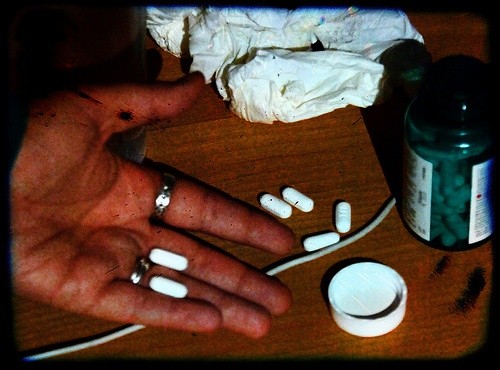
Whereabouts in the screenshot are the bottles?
[402,52,499,249]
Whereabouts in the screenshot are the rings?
[150,172,176,224]
[128,257,151,285]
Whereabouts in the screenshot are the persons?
[0,70,295,340]
